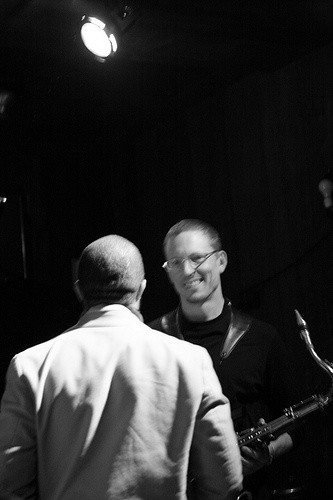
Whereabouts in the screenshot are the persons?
[144,221,333,500]
[0,235,247,500]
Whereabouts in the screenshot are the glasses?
[162,250,219,273]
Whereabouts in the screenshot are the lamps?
[80,0,137,65]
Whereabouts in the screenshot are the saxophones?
[234,309,332,449]
